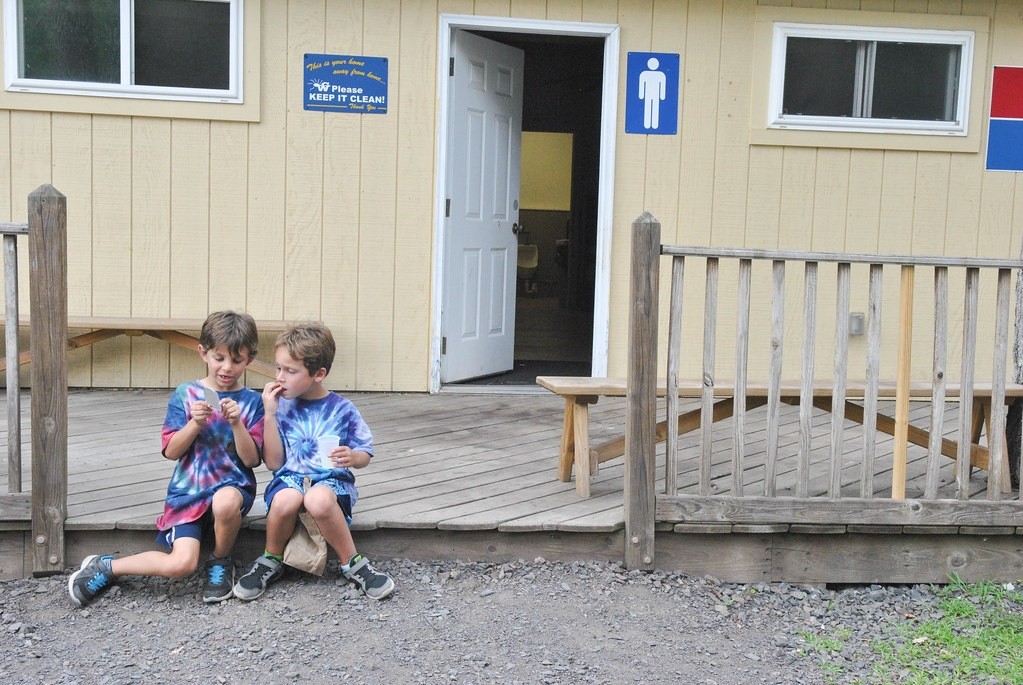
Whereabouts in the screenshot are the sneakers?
[203,556,236,602]
[69,553,117,607]
[339,554,393,600]
[233,559,285,601]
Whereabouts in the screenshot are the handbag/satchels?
[281,476,328,576]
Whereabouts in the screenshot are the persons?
[232,315,397,603]
[67,309,266,609]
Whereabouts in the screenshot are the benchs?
[0,314,325,388]
[536,373,1023,506]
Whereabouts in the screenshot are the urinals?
[552,238,575,281]
[516,244,539,281]
